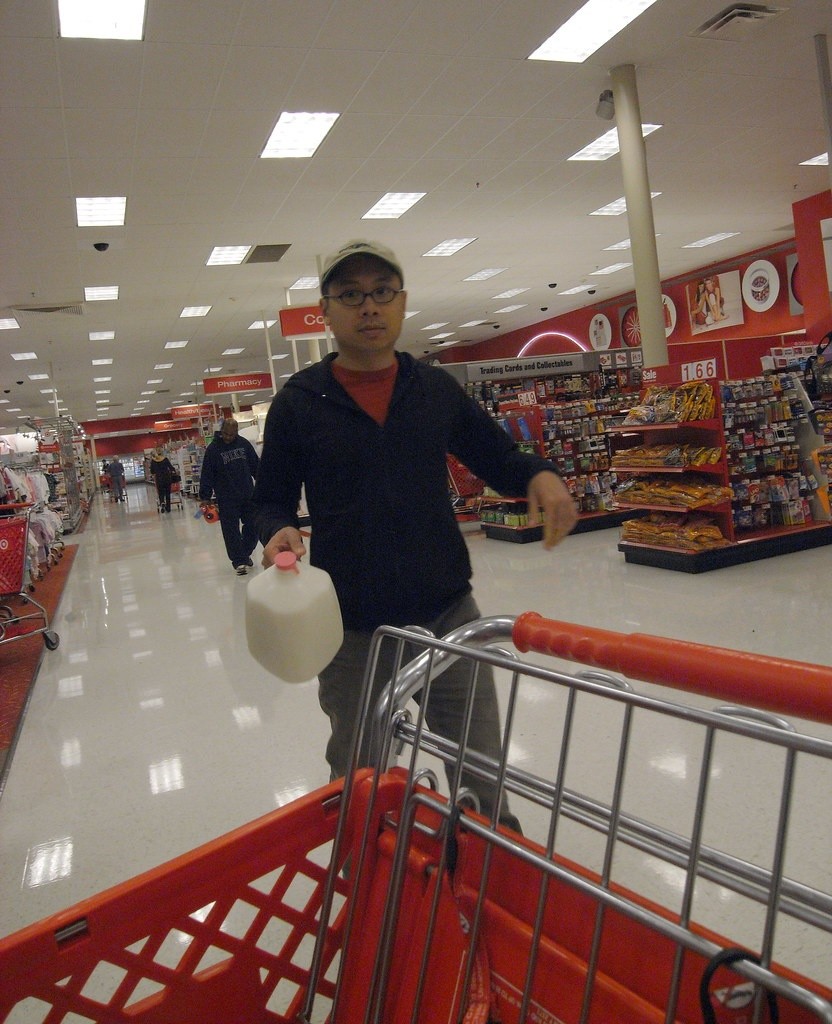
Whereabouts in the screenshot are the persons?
[149,447,175,513]
[690,277,729,330]
[199,418,260,576]
[102,459,109,493]
[107,455,125,503]
[249,238,579,880]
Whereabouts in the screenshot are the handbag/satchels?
[170,469,181,483]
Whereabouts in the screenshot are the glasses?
[324,286,404,306]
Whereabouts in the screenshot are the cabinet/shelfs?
[143,441,206,503]
[34,429,95,530]
[479,405,546,545]
[96,452,147,486]
[603,377,736,574]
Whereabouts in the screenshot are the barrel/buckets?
[244,551,343,684]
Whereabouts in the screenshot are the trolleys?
[0,614,832,1023]
[153,470,185,514]
[98,469,128,502]
[0,500,61,652]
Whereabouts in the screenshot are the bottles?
[480,506,544,526]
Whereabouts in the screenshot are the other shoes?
[161,503,165,513]
[235,563,247,575]
[121,499,125,501]
[165,507,170,512]
[115,500,118,502]
[246,556,254,568]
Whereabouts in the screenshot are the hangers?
[0,461,57,519]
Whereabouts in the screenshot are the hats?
[317,239,404,295]
